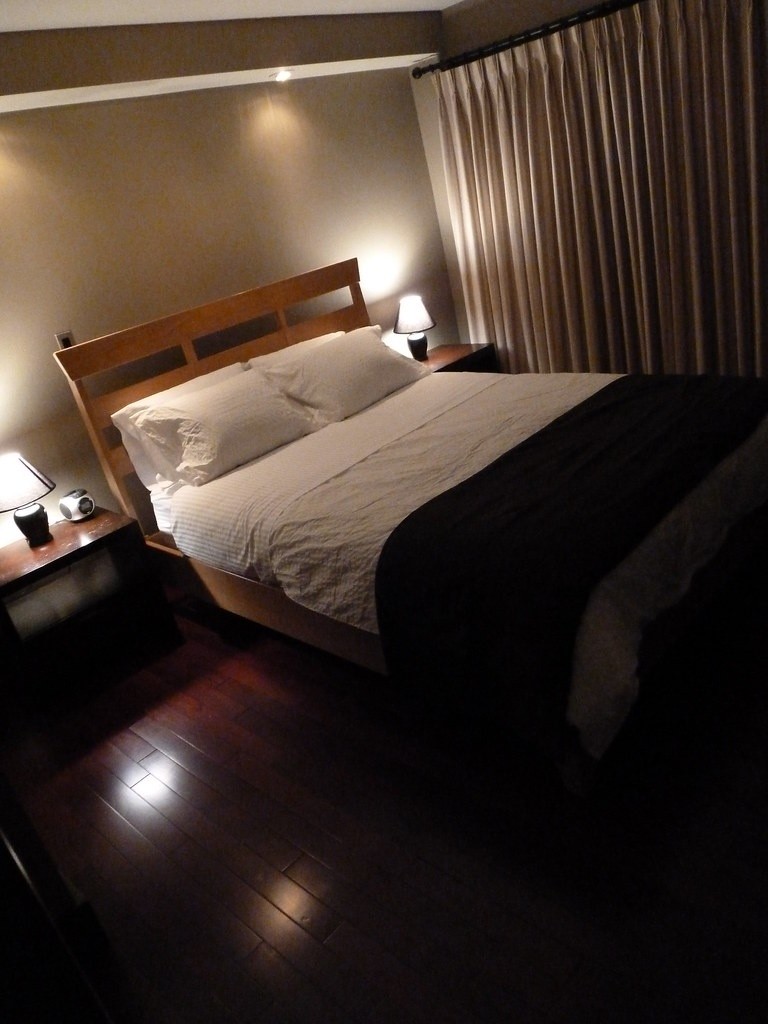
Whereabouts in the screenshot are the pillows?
[111,324,433,488]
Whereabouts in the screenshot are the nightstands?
[0,506,187,722]
[422,343,499,373]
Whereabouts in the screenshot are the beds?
[58,258,768,763]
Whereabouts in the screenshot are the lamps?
[394,293,436,362]
[0,452,56,550]
